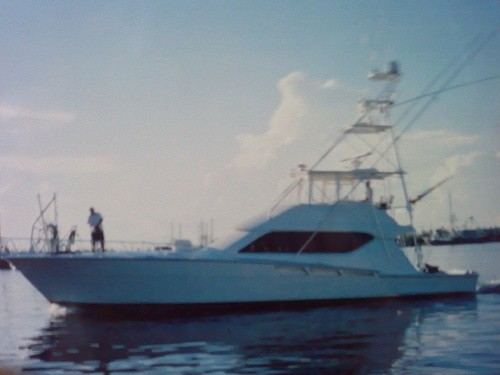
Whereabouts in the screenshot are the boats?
[1,56,482,309]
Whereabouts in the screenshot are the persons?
[87,206,106,253]
